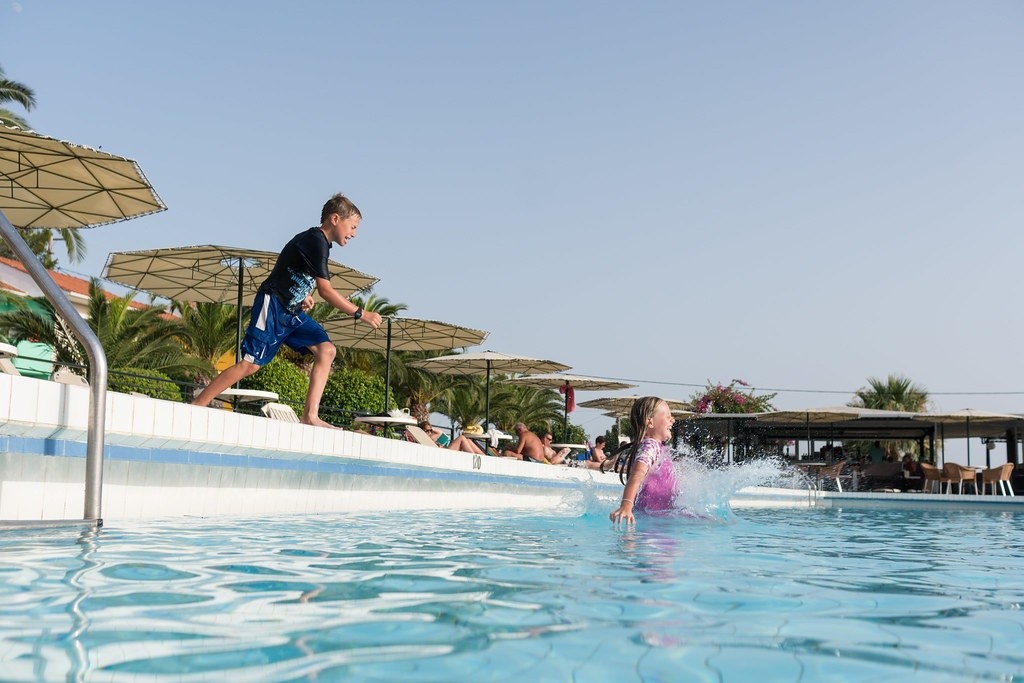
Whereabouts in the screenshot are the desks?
[192,387,280,412]
[795,462,827,489]
[353,415,418,438]
[964,465,988,495]
[461,433,513,456]
[547,443,588,467]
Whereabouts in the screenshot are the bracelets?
[621,499,635,507]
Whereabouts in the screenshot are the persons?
[827,440,925,493]
[535,428,619,471]
[421,420,489,457]
[193,192,383,431]
[593,436,634,474]
[600,396,682,525]
[505,424,546,464]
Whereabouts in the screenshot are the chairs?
[982,462,1018,496]
[259,402,302,423]
[403,424,441,448]
[919,462,942,495]
[943,462,979,495]
[816,461,847,492]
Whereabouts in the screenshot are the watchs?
[355,308,363,322]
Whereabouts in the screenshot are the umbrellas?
[577,396,706,451]
[314,316,490,440]
[0,124,169,230]
[100,245,382,412]
[755,410,859,490]
[495,375,642,466]
[406,349,573,458]
[914,409,1024,496]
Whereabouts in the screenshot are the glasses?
[543,437,552,441]
[423,428,433,432]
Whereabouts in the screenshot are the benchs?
[859,462,908,494]
